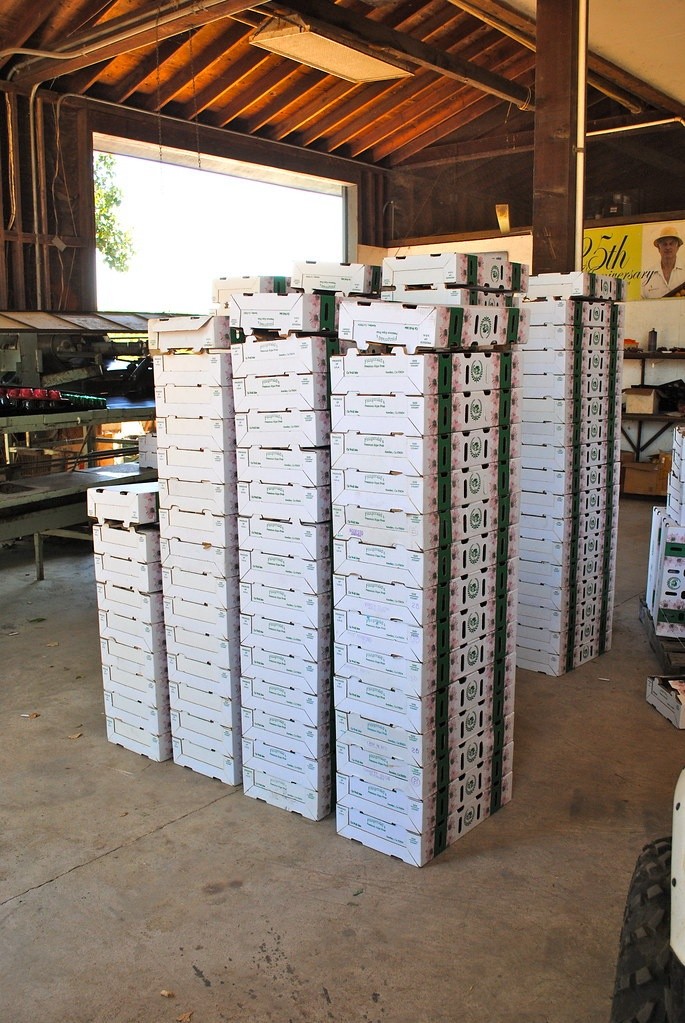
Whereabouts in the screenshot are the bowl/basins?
[624,339,639,352]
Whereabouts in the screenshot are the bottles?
[647,328,657,352]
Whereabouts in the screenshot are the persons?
[640,225,685,299]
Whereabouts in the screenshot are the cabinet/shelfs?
[622,331,682,503]
[0,307,79,581]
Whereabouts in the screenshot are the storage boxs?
[621,449,635,467]
[78,243,630,866]
[658,448,674,496]
[625,387,660,415]
[640,427,684,733]
[622,461,658,496]
